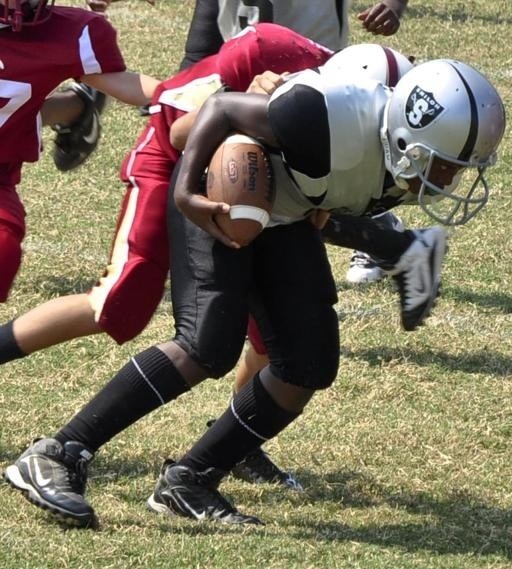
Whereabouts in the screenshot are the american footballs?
[207,133,276,247]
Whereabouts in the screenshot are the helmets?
[380,58,506,226]
[0,0,54,32]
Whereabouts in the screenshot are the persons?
[0,0,163,301]
[1,19,417,485]
[179,0,452,335]
[1,57,508,533]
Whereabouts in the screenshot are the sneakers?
[1,437,94,526]
[54,81,108,171]
[383,226,449,333]
[144,459,261,527]
[228,447,303,491]
[347,211,405,283]
